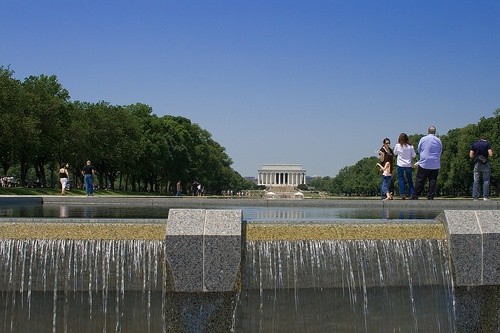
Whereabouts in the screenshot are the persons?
[176,179,205,196]
[410,126,443,200]
[469,136,493,200]
[59,163,68,194]
[226,189,245,196]
[82,160,94,196]
[374,156,392,199]
[1,174,41,190]
[393,133,417,200]
[378,137,394,201]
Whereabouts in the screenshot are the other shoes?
[61,194,65,195]
[474,197,479,201]
[483,197,492,201]
[401,196,418,200]
[87,193,93,196]
[429,198,433,200]
[384,196,394,201]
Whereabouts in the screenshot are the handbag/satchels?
[478,155,487,164]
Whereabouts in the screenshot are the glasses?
[385,142,390,144]
